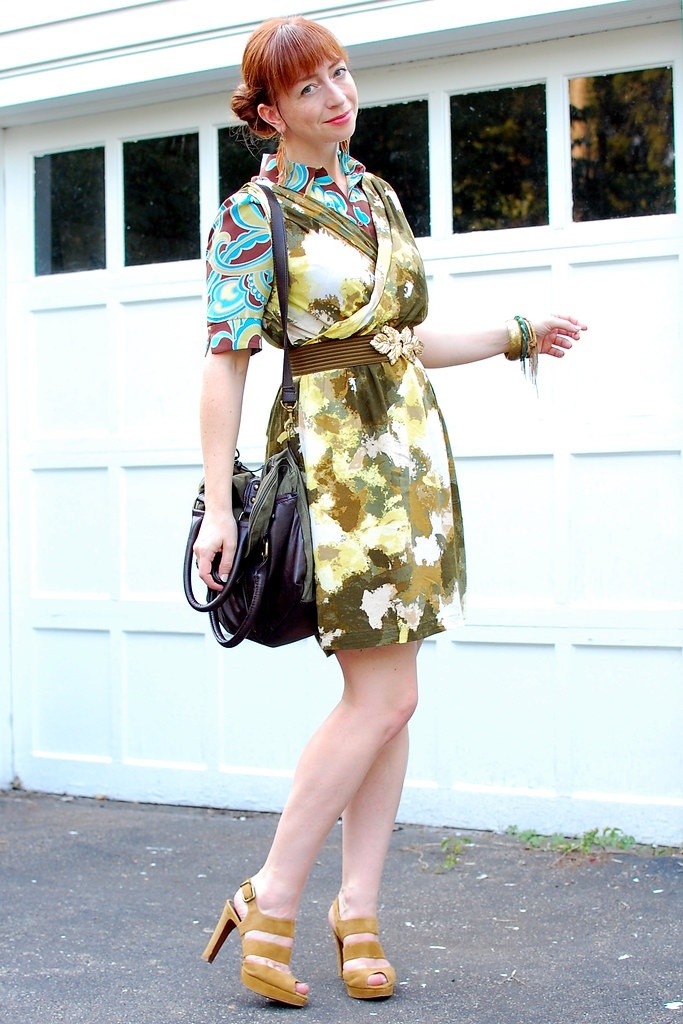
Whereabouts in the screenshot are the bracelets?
[504,315,538,379]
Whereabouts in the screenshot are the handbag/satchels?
[184,449,316,648]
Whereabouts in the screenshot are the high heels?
[201,879,309,1007]
[328,897,395,999]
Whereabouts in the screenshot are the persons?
[201,17,588,1007]
